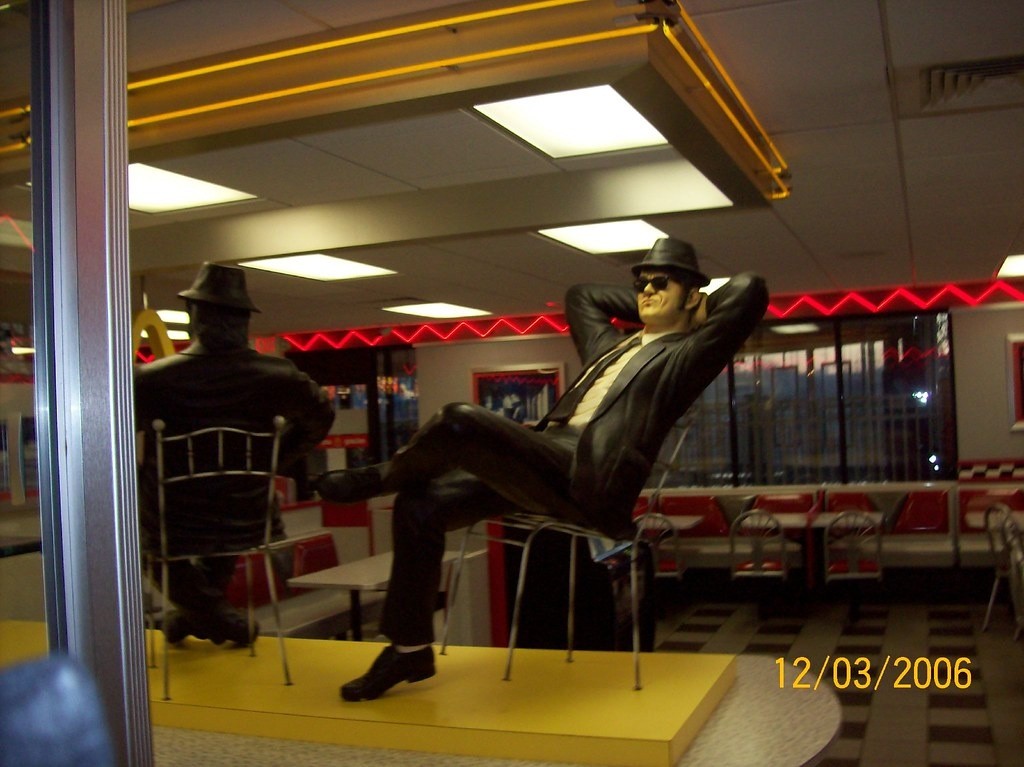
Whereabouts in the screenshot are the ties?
[547,337,641,424]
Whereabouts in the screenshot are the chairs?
[824,511,886,619]
[730,510,789,618]
[145,414,291,700]
[633,513,687,618]
[439,404,701,694]
[978,505,1024,636]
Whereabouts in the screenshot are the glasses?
[632,276,668,290]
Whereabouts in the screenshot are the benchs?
[956,485,1024,566]
[228,531,386,641]
[824,486,955,568]
[659,489,818,569]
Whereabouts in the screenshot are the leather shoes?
[340,646,438,702]
[238,619,259,645]
[315,462,396,504]
[162,617,185,644]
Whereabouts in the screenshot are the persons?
[480,391,525,424]
[133,261,336,648]
[316,239,770,701]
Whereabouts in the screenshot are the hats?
[630,237,710,287]
[177,262,262,314]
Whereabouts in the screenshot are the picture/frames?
[1006,333,1024,433]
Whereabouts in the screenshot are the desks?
[811,513,884,609]
[966,511,1024,632]
[637,516,704,609]
[741,513,808,616]
[288,549,465,642]
